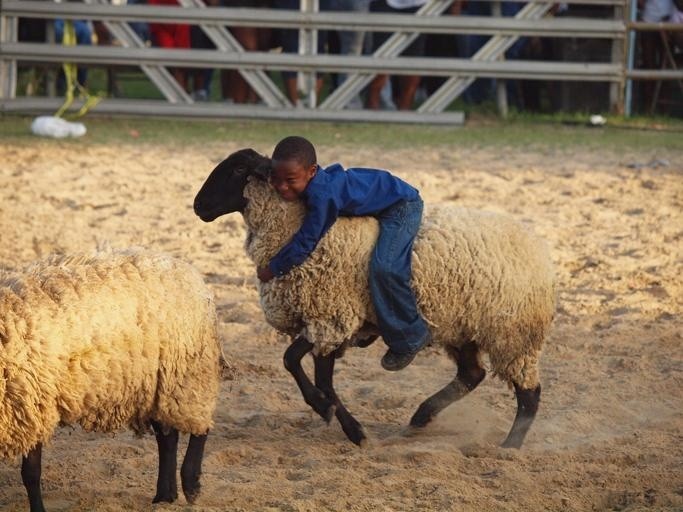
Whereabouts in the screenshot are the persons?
[18,0,683,113]
[257,136,431,371]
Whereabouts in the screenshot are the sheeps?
[1,246,222,512]
[192,147,557,450]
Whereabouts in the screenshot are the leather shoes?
[380,328,432,371]
